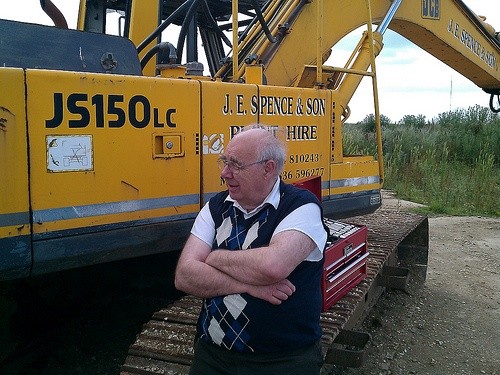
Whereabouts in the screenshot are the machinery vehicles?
[0,1,500,375]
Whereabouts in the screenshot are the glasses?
[216,156,268,175]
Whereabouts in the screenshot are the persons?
[174,125,330,375]
